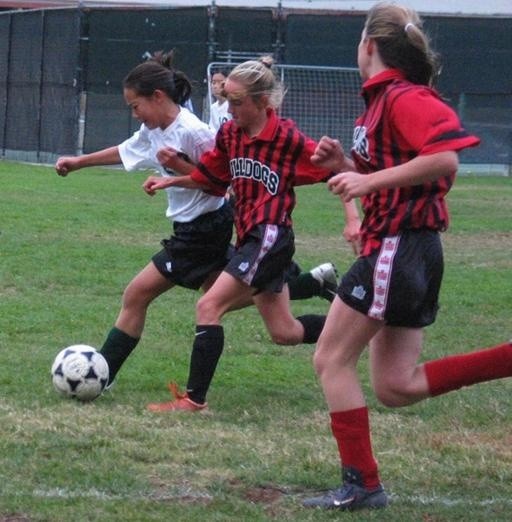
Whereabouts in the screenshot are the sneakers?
[310,261,339,303]
[146,384,208,413]
[300,471,386,510]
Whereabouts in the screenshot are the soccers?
[51,345,110,402]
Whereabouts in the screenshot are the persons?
[55,61,338,390]
[300,2,512,511]
[141,56,363,415]
[209,67,233,134]
